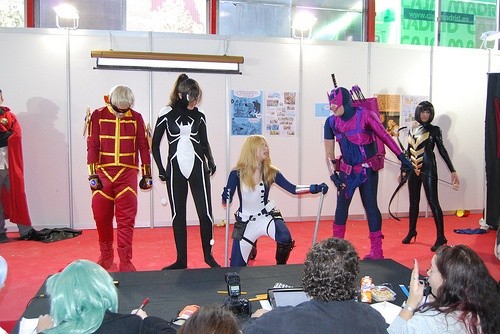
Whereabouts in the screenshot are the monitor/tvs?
[268,288,313,308]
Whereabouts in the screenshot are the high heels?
[402,230,417,243]
[430,238,448,250]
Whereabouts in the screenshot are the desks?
[11,258,426,334]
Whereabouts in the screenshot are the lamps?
[479,31,500,51]
[292,12,317,39]
[52,2,80,29]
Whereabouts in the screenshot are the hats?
[329,88,351,105]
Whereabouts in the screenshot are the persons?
[397,101,460,252]
[0,89,36,243]
[0,255,240,334]
[390,224,500,334]
[221,135,328,268]
[152,74,221,270]
[241,238,390,334]
[324,87,413,260]
[87,85,152,272]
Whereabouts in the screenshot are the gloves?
[317,183,329,194]
[139,176,153,189]
[222,187,229,203]
[88,175,104,190]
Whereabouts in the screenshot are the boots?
[363,231,385,260]
[332,221,346,239]
[249,240,257,259]
[276,239,296,264]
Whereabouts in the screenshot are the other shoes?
[22,229,40,241]
[0,233,9,242]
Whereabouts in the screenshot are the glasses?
[186,94,199,102]
[110,99,130,113]
